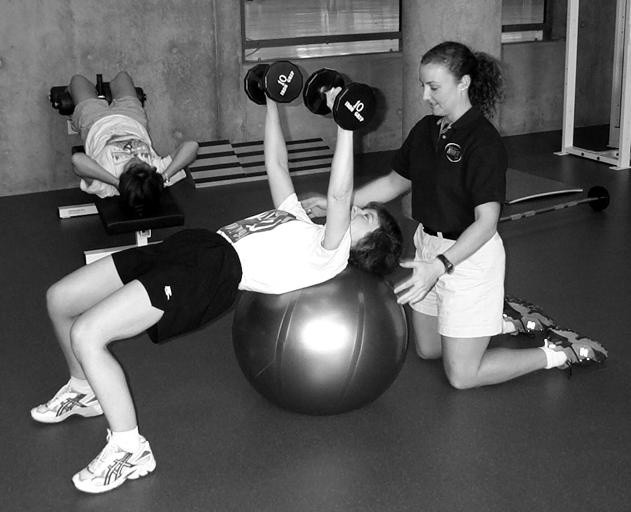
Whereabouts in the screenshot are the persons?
[296,40,609,388]
[27,83,407,493]
[65,70,201,212]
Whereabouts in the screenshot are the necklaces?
[440,116,456,135]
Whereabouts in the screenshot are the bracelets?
[163,170,172,183]
[438,252,454,274]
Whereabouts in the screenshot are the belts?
[422,225,461,241]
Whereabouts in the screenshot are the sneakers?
[71,438,157,494]
[502,296,557,335]
[30,381,106,424]
[543,324,609,375]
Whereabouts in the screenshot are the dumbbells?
[244,59,302,105]
[303,66,376,131]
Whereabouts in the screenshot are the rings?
[305,208,314,216]
